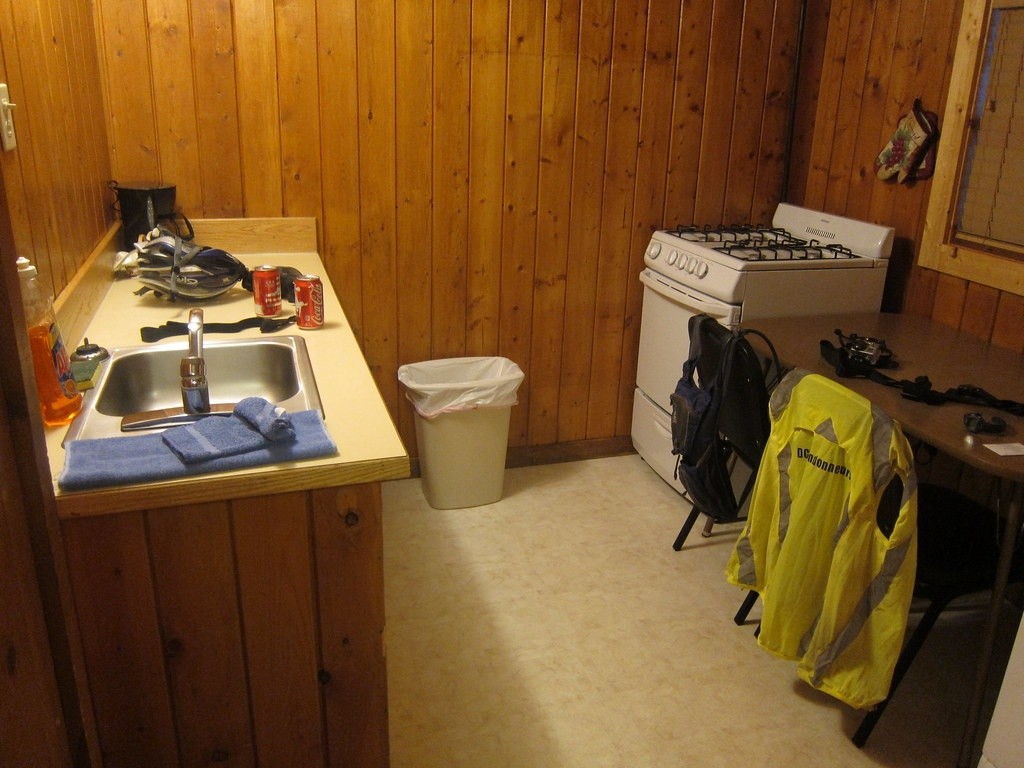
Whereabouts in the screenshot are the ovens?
[627,239,748,498]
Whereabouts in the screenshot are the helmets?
[133,226,246,302]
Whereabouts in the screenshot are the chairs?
[670,315,794,551]
[735,369,1003,749]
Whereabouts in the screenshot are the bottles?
[16,256,84,426]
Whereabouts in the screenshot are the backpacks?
[665,314,782,524]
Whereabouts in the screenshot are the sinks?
[60,334,325,449]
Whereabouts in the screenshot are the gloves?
[875,99,939,185]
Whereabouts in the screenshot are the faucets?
[177,308,210,413]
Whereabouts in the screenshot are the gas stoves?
[651,222,875,271]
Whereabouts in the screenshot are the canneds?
[252,265,283,318]
[293,275,324,329]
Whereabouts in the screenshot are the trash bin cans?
[397,355,525,510]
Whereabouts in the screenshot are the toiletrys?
[16,254,84,427]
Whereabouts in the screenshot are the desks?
[739,308,1023,768]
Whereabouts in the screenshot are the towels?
[161,395,296,465]
[56,409,337,492]
